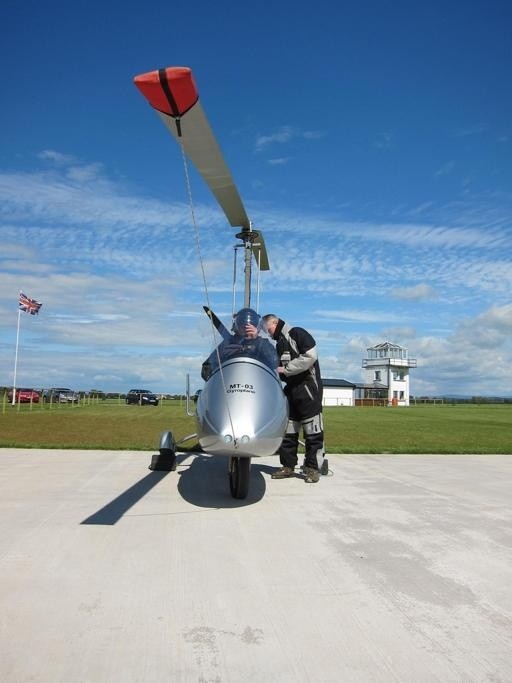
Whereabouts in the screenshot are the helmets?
[232,308,261,336]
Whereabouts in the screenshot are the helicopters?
[133,66,290,502]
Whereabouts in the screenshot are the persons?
[260,312,324,484]
[199,307,280,384]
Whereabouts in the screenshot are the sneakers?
[306,468,319,483]
[271,466,294,480]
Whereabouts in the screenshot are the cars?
[41,386,81,404]
[125,389,159,408]
[6,387,40,404]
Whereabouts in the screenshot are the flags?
[19,293,43,316]
